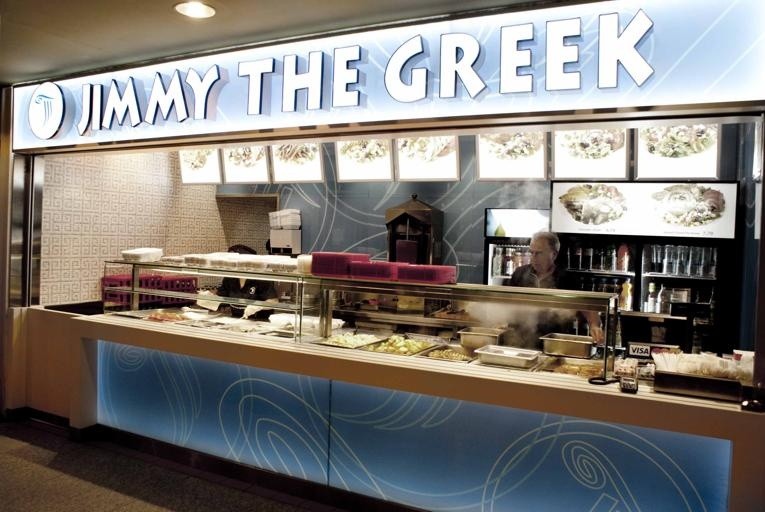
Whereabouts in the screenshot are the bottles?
[578,276,634,311]
[567,244,630,273]
[644,282,669,313]
[491,247,531,277]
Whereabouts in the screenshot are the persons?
[211,243,279,322]
[508,230,604,354]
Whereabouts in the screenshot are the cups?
[649,244,717,278]
